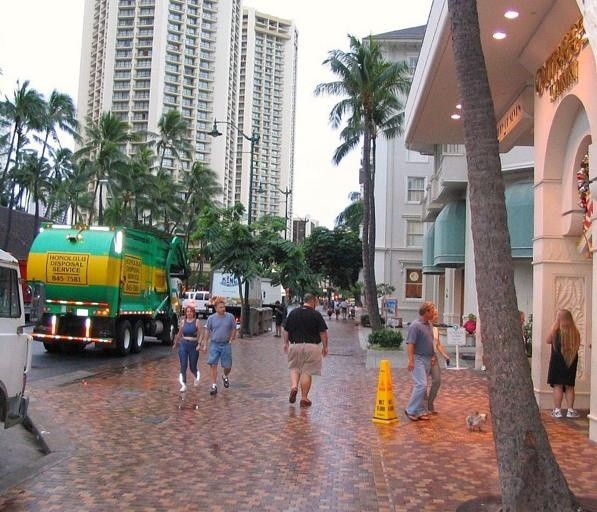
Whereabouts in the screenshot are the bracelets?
[197,342,201,345]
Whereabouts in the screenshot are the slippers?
[405,411,430,421]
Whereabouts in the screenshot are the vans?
[183,291,209,320]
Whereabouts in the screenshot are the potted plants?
[366,327,411,370]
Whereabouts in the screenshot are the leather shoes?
[289,388,298,403]
[300,399,311,407]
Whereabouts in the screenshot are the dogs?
[465,410,488,432]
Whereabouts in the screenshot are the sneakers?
[180,375,229,394]
[567,411,581,418]
[551,411,563,418]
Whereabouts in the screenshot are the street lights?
[207,117,261,337]
[301,216,309,240]
[255,182,291,239]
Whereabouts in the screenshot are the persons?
[171,305,203,393]
[424,306,452,415]
[202,300,237,395]
[318,294,356,321]
[282,303,287,327]
[519,310,529,354]
[404,300,438,422]
[273,300,284,336]
[546,308,581,418]
[280,292,329,411]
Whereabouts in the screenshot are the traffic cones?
[369,358,400,425]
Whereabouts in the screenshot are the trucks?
[26,221,189,356]
[206,269,283,318]
[0,250,47,429]
